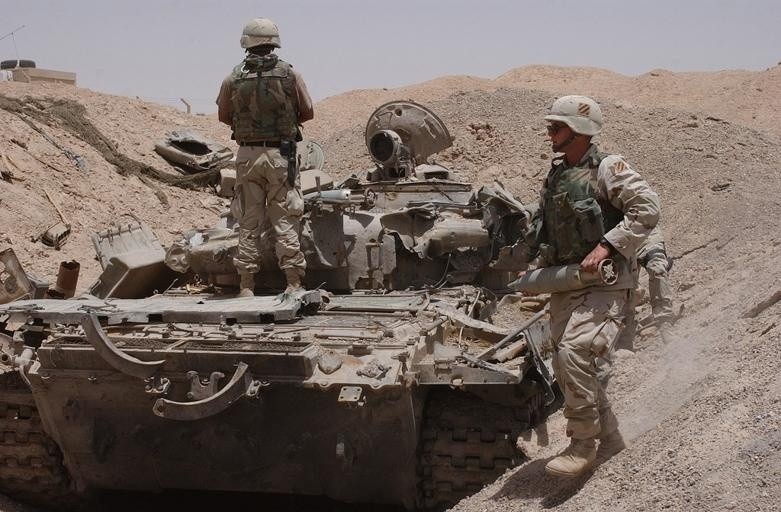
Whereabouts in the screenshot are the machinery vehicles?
[1,100,565,512]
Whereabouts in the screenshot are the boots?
[592,429,625,466]
[660,322,677,345]
[236,274,254,297]
[545,437,596,478]
[284,269,302,293]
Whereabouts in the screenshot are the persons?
[631,222,674,346]
[215,19,318,296]
[518,89,660,475]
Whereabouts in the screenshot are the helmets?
[544,95,603,136]
[240,19,281,48]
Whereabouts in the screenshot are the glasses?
[547,126,569,134]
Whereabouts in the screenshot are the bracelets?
[599,236,615,253]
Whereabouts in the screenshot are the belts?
[240,141,281,147]
